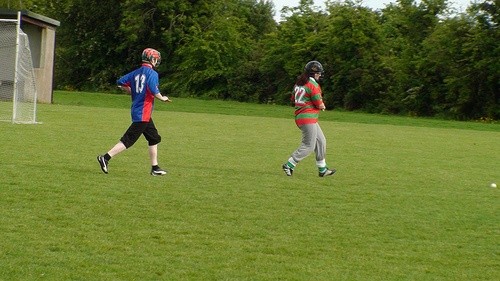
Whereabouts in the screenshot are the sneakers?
[282,164,293,176]
[97,155,109,174]
[150,169,168,177]
[318,168,336,177]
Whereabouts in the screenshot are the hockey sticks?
[118,86,172,102]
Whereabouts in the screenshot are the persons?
[282,60,337,178]
[96,47,169,177]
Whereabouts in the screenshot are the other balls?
[491,183,496,188]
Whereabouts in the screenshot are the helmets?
[141,48,161,68]
[304,61,324,73]
